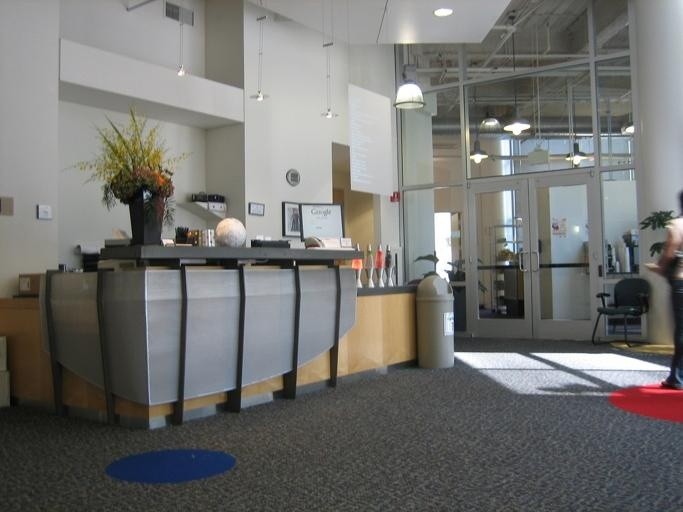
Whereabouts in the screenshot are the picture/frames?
[281,201,345,242]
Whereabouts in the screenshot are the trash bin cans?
[416,275,455,369]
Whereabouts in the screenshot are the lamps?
[564,80,591,168]
[392,43,426,111]
[470,9,531,164]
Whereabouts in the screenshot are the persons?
[290,210,300,230]
[649,189,683,390]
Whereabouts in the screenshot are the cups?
[202,229,215,246]
[612,238,631,273]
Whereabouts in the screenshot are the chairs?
[591,279,649,347]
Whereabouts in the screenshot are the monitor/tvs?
[251,239,290,247]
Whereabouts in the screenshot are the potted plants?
[68,103,193,245]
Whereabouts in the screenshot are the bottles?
[192,192,227,212]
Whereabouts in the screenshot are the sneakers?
[667,368,682,385]
